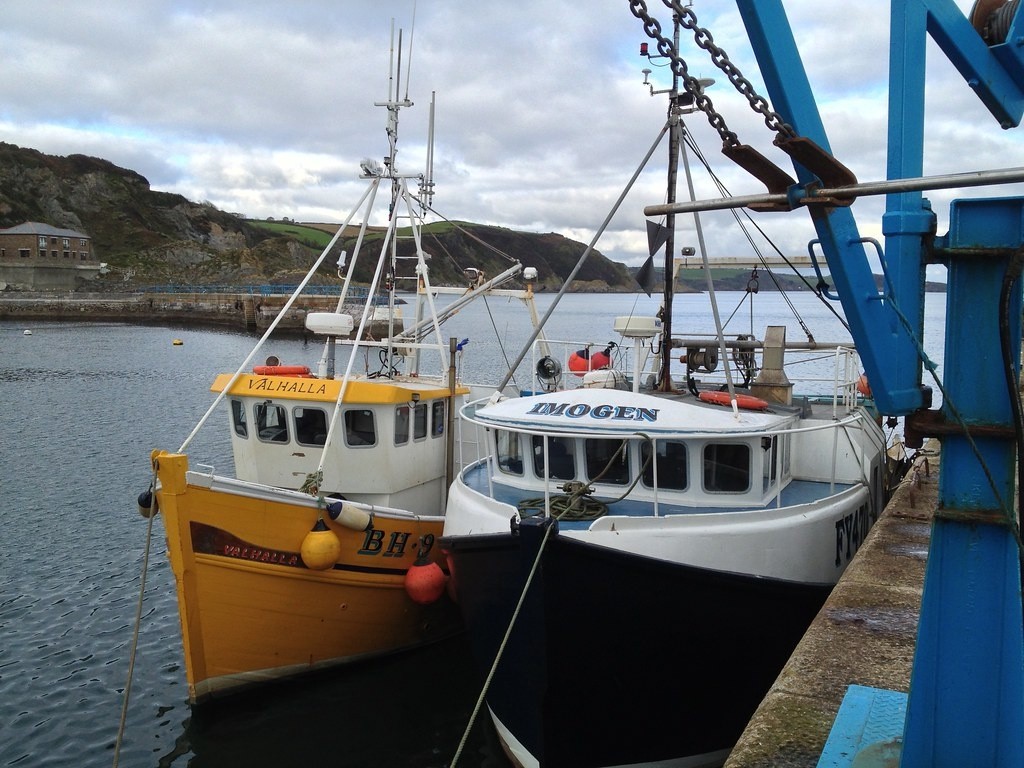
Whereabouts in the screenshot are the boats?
[138,10,556,709]
[446,4,896,763]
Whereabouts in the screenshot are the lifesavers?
[253,365,317,381]
[699,389,769,411]
